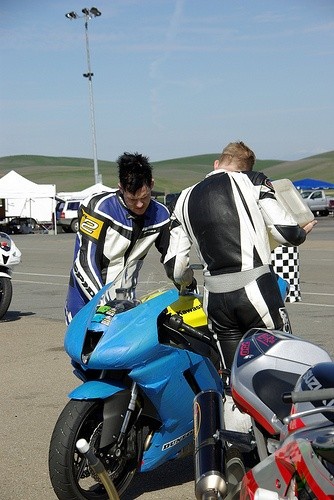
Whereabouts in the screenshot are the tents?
[291,179,334,190]
[0,184,57,235]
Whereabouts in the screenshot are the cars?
[0,215,37,234]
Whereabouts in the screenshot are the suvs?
[49,195,90,233]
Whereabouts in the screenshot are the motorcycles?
[0,231,22,319]
[48,261,293,500]
[187,324,333,500]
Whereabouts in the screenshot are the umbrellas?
[163,139,319,392]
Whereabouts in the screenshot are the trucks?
[298,189,334,216]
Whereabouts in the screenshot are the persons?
[64,153,199,384]
